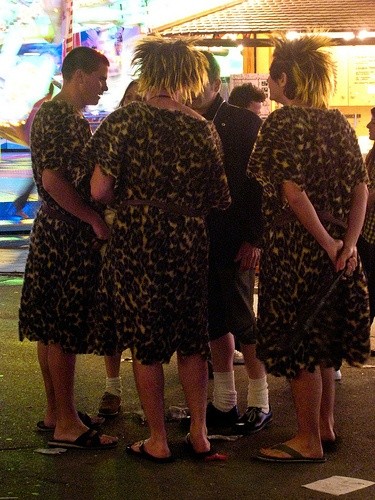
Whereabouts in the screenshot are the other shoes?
[233,351,243,365]
[100,393,123,415]
[334,371,342,382]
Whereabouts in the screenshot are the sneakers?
[180,406,239,442]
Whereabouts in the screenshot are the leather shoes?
[237,407,273,433]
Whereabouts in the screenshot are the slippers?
[126,438,171,463]
[255,439,326,464]
[36,412,90,433]
[50,433,119,449]
[318,434,340,451]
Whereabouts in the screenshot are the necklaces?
[148,94,173,101]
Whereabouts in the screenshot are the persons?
[91,39,224,461]
[98,78,143,424]
[18,47,115,447]
[179,49,274,443]
[248,31,369,462]
[227,82,266,117]
[358,106,374,369]
